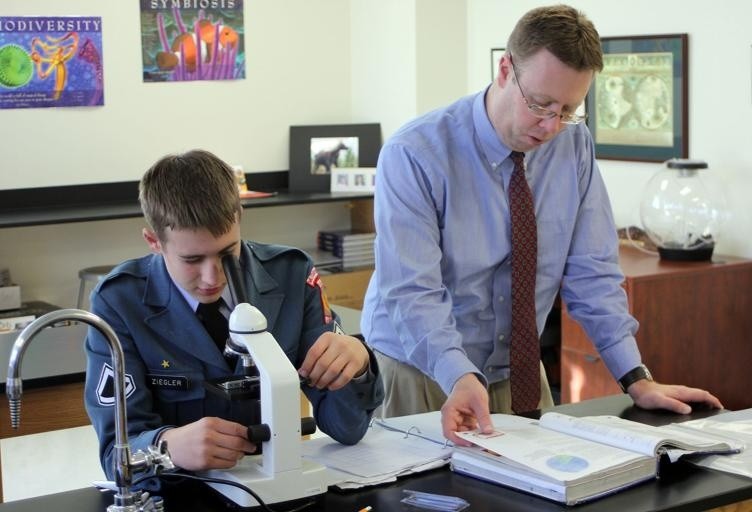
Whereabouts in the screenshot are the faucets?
[5,308,133,495]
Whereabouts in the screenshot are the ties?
[197,297,239,373]
[509,150,541,417]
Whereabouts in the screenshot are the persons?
[83,150,385,492]
[360,4,724,448]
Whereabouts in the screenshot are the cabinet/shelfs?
[560,243,752,408]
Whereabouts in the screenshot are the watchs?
[618,365,654,394]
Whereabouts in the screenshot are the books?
[449,411,741,508]
[317,228,376,271]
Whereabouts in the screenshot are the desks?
[0,184,377,234]
[2,390,751,510]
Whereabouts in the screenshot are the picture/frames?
[287,122,383,196]
[489,33,691,166]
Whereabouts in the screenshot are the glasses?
[508,53,591,126]
